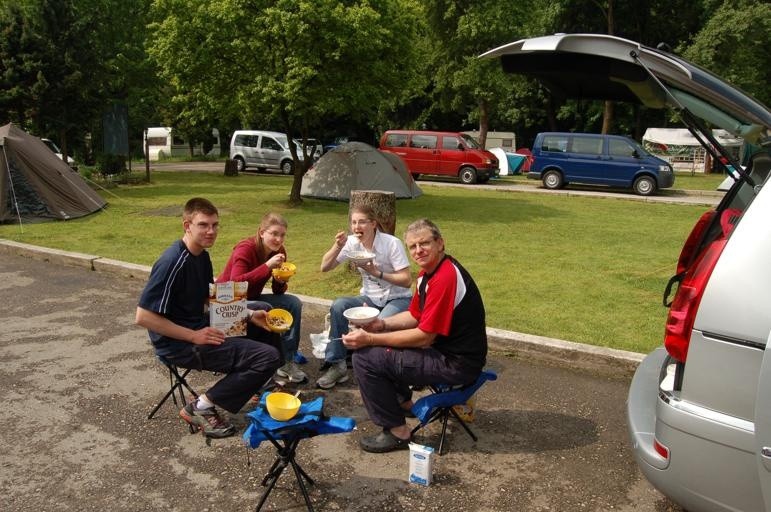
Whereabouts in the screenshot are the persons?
[315,203,415,390]
[133,197,286,439]
[338,216,489,454]
[213,213,311,385]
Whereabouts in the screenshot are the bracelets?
[380,271,384,279]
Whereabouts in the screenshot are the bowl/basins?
[272,262,296,278]
[345,250,376,266]
[343,306,381,325]
[265,392,301,422]
[266,309,293,333]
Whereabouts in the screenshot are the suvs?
[477,31,771,511]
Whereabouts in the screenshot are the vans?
[295,138,343,161]
[229,129,304,175]
[527,131,677,195]
[379,129,500,185]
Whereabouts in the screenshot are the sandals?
[357,429,413,452]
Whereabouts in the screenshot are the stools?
[145,349,208,435]
[242,395,355,512]
[406,368,499,459]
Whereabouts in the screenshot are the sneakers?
[180,399,236,438]
[316,366,350,389]
[276,362,310,383]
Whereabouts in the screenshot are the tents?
[287,139,426,201]
[1,121,131,236]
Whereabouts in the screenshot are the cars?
[40,138,78,173]
